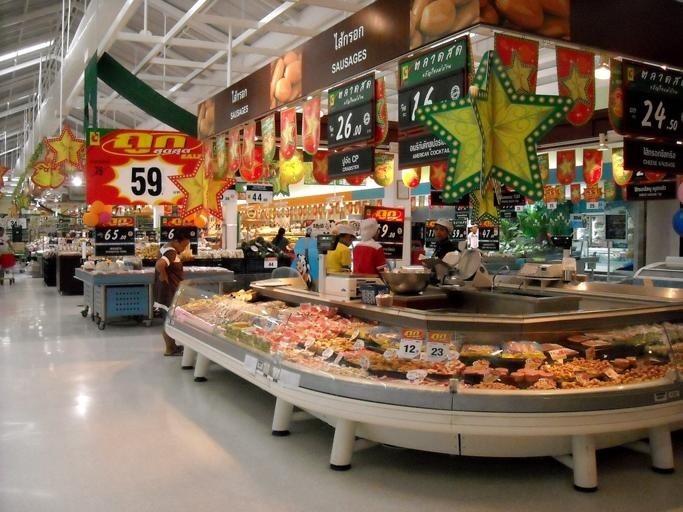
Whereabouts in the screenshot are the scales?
[520,235,576,278]
[318,234,390,300]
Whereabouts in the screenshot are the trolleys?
[6,250,25,274]
[80,280,94,321]
[0,249,15,285]
[93,283,152,330]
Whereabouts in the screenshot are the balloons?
[672,182,683,237]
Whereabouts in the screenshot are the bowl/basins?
[376,270,431,297]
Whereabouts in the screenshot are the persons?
[153,235,191,357]
[353,217,389,275]
[429,217,459,259]
[0,225,16,289]
[271,225,295,252]
[326,223,359,275]
[466,219,479,249]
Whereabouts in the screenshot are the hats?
[360,218,379,240]
[337,224,357,238]
[432,218,451,232]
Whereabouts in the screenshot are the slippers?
[164,346,183,356]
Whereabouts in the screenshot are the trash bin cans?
[32,265,41,278]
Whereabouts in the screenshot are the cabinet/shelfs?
[162,287,681,493]
[43,250,292,327]
